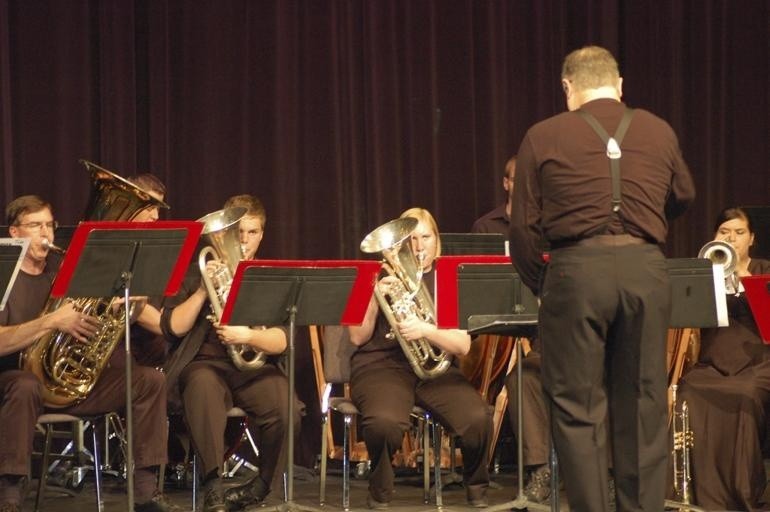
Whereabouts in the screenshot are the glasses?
[15,220,58,233]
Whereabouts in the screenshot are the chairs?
[319,326,444,512]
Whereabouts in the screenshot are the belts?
[578,233,642,246]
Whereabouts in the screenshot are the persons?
[508,321,565,508]
[347,208,495,511]
[470,155,519,256]
[507,45,695,512]
[125,173,167,366]
[674,205,770,512]
[160,195,301,512]
[0,195,184,512]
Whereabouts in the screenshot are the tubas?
[194,206,271,371]
[23,158,170,409]
[360,217,453,380]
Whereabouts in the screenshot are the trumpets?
[698,240,738,294]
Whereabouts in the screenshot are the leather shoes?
[466,488,488,509]
[367,478,394,503]
[223,476,272,512]
[514,465,563,511]
[201,487,226,512]
[0,478,24,512]
[134,493,190,512]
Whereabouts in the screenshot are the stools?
[170,405,263,506]
[26,411,105,512]
